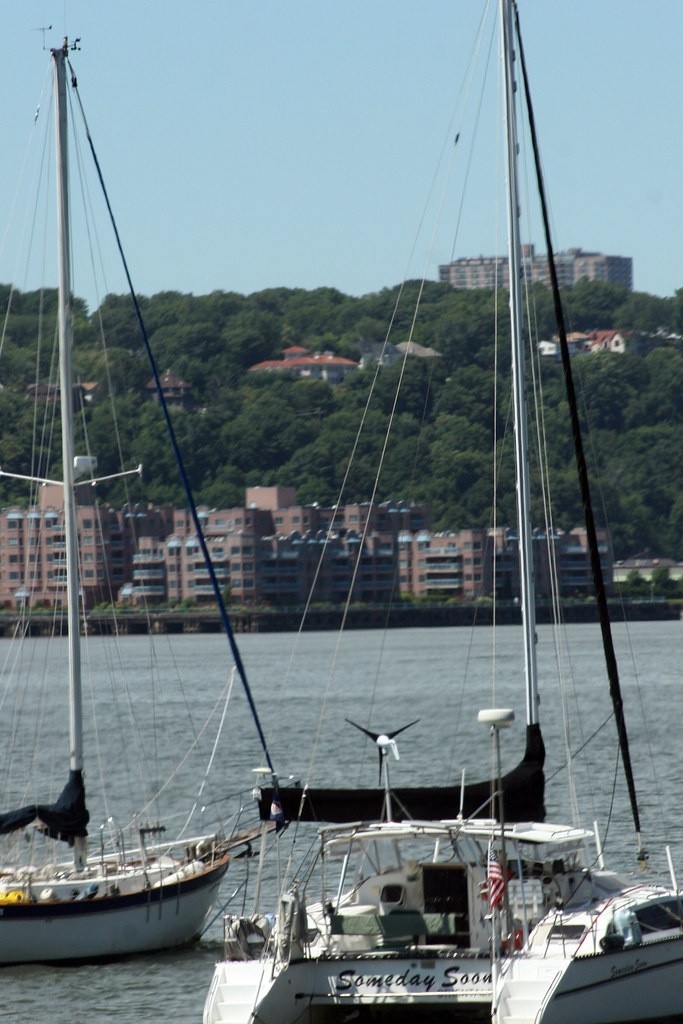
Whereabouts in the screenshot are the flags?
[269,784,286,839]
[487,840,505,912]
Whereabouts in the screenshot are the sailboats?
[2,0,683,1024]
